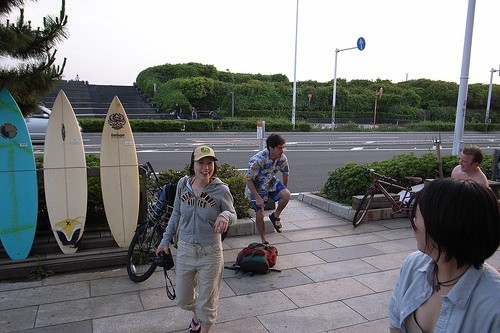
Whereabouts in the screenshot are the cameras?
[153,248,174,270]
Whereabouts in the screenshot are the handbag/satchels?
[239,256,269,274]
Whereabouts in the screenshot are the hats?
[193,146,218,161]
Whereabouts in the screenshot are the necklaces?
[433,262,473,292]
[190,177,211,189]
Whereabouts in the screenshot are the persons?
[388,178,500,333]
[156,145,237,333]
[451,146,488,188]
[245,133,291,245]
[191,107,200,120]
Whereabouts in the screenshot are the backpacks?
[231,242,277,270]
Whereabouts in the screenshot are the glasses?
[276,146,285,151]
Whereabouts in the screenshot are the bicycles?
[353,167,423,227]
[126,161,230,283]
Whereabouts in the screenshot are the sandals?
[189,319,201,333]
[269,212,282,233]
[263,241,269,245]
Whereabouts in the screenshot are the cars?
[24,103,82,140]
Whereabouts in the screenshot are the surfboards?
[0,84,40,264]
[41,88,90,256]
[99,95,141,251]
